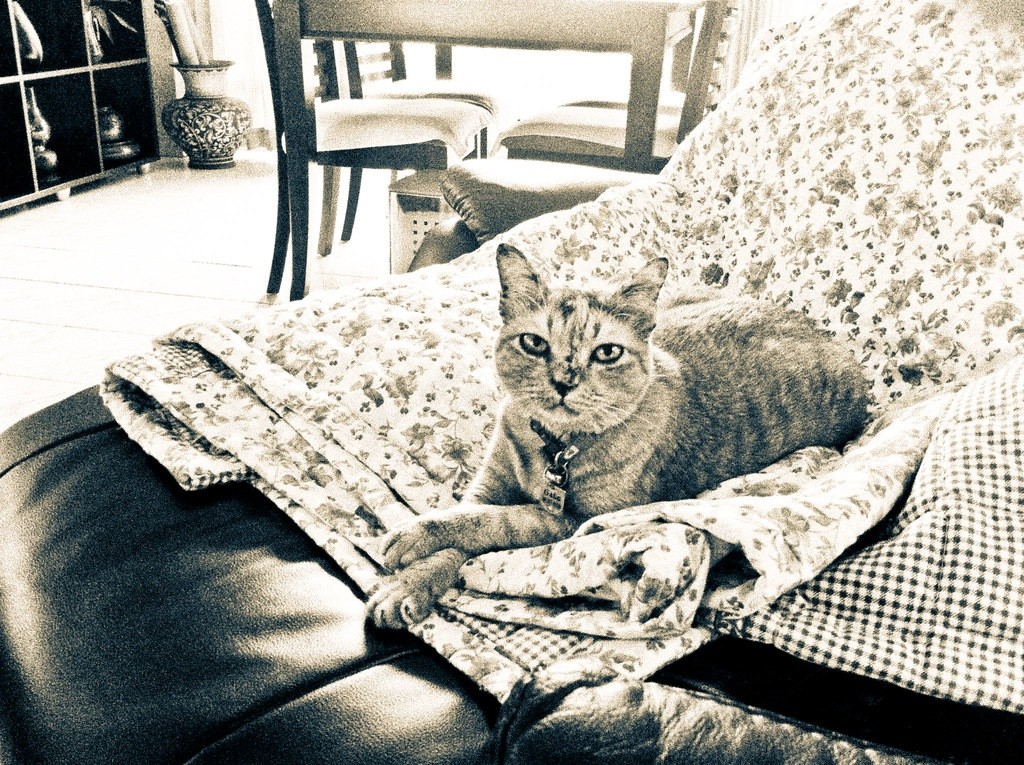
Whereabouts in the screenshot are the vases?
[162,61,253,171]
[26,87,58,188]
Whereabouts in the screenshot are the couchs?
[0,0,1024,765]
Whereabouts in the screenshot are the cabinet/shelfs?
[0,0,163,212]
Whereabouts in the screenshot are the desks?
[274,0,705,302]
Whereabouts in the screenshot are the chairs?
[256,0,493,293]
[340,37,500,244]
[502,0,742,175]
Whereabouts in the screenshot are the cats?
[365,242,869,630]
